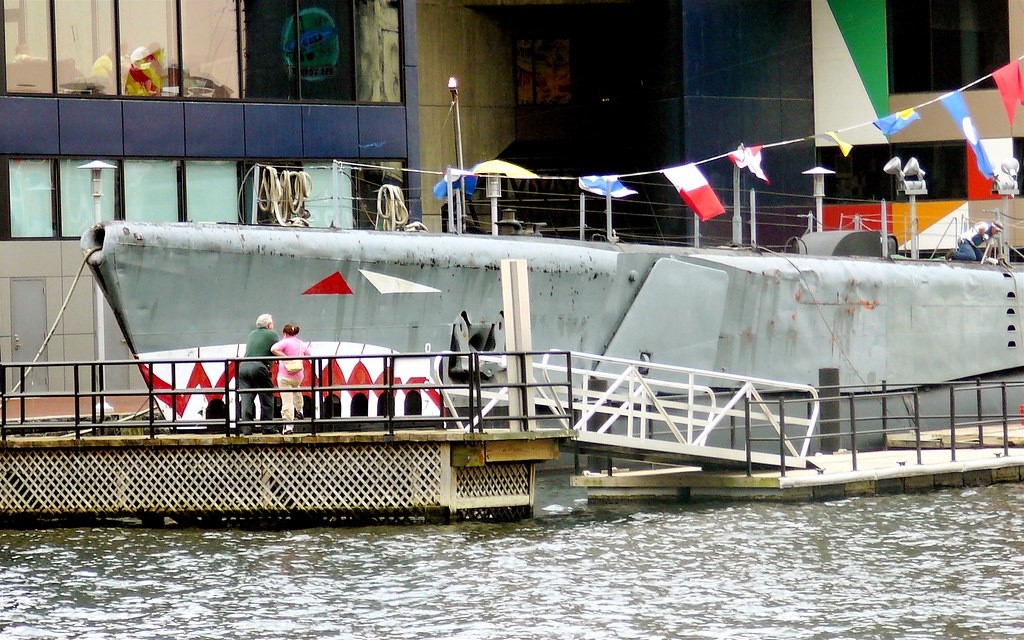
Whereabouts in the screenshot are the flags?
[578,174,639,198]
[816,131,854,157]
[939,90,994,180]
[873,108,921,136]
[434,168,478,199]
[661,164,725,222]
[729,143,771,184]
[991,59,1024,129]
[472,159,538,178]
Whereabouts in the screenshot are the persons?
[90,42,162,95]
[271,324,312,435]
[945,220,1004,261]
[238,313,279,434]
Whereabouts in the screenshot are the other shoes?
[297,412,304,420]
[264,429,280,434]
[285,430,293,434]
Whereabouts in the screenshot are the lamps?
[1001,157,1020,189]
[902,157,926,181]
[883,157,910,190]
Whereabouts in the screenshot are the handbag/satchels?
[283,360,303,374]
